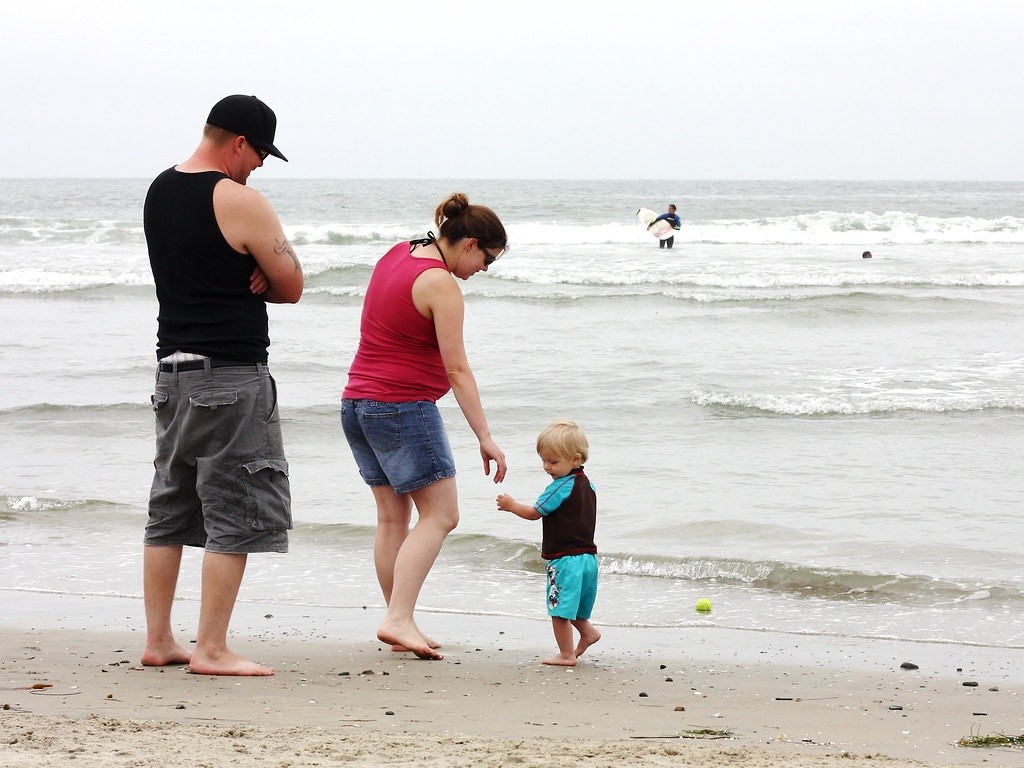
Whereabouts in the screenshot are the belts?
[159,357,267,373]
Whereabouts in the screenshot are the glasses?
[465,234,495,266]
[247,140,269,160]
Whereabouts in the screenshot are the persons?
[496,421,602,666]
[341,192,512,662]
[137,94,305,676]
[863,251,871,258]
[647,204,681,249]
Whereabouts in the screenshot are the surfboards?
[636,208,675,241]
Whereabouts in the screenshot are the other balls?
[694,597,712,612]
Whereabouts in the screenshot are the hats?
[206,95,287,162]
